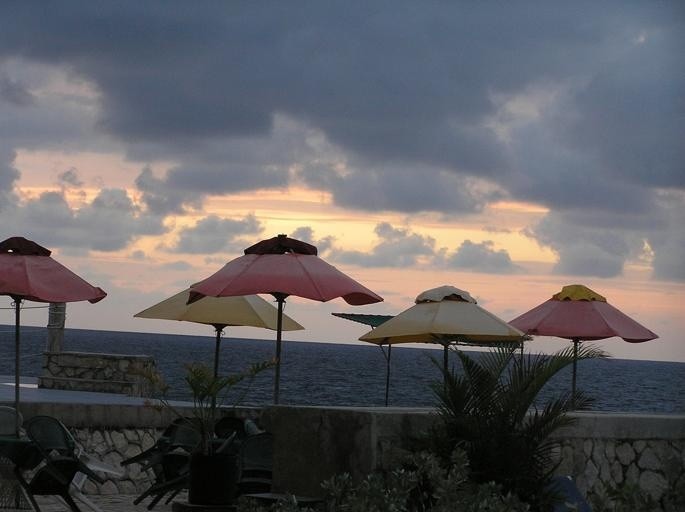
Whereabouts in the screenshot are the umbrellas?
[331,305,403,405]
[133,282,306,424]
[0,232,106,435]
[188,233,387,402]
[358,283,536,402]
[508,283,659,409]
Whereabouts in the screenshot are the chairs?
[0,403,270,512]
